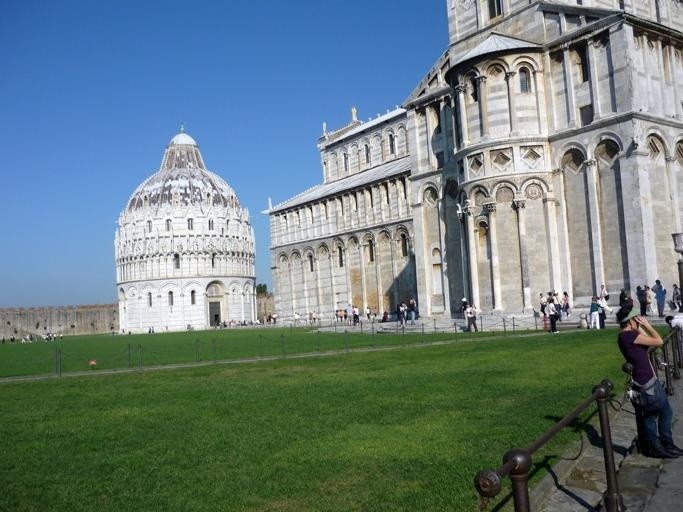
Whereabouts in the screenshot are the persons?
[540,291,570,334]
[590,280,683,330]
[335,305,388,326]
[295,311,316,324]
[214,312,278,330]
[616,305,683,458]
[397,297,417,326]
[2,333,63,344]
[111,324,154,336]
[461,297,478,332]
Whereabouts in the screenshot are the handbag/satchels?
[630,374,668,415]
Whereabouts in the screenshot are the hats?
[621,308,639,323]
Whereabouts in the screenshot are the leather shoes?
[649,443,682,458]
